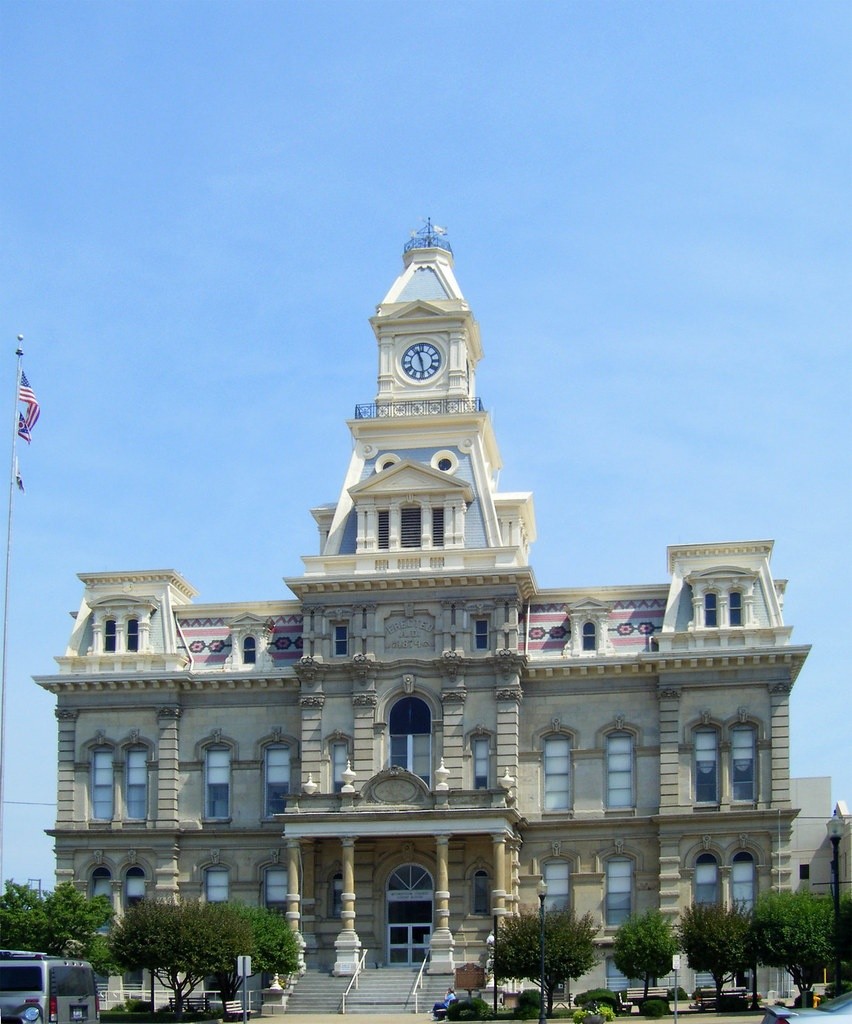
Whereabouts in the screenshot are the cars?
[762,991,852,1024]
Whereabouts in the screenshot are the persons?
[427,988,456,1021]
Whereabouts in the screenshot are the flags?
[16,358,40,489]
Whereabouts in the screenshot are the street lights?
[536,879,548,1024]
[826,809,844,997]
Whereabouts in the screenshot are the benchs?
[169,998,210,1012]
[225,1001,251,1021]
[436,999,460,1019]
[618,987,667,1014]
[544,993,573,1010]
[696,986,747,1011]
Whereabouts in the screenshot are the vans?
[0,950,100,1024]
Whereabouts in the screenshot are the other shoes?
[432,1017,438,1021]
[427,1009,434,1013]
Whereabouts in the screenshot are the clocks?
[401,342,442,379]
